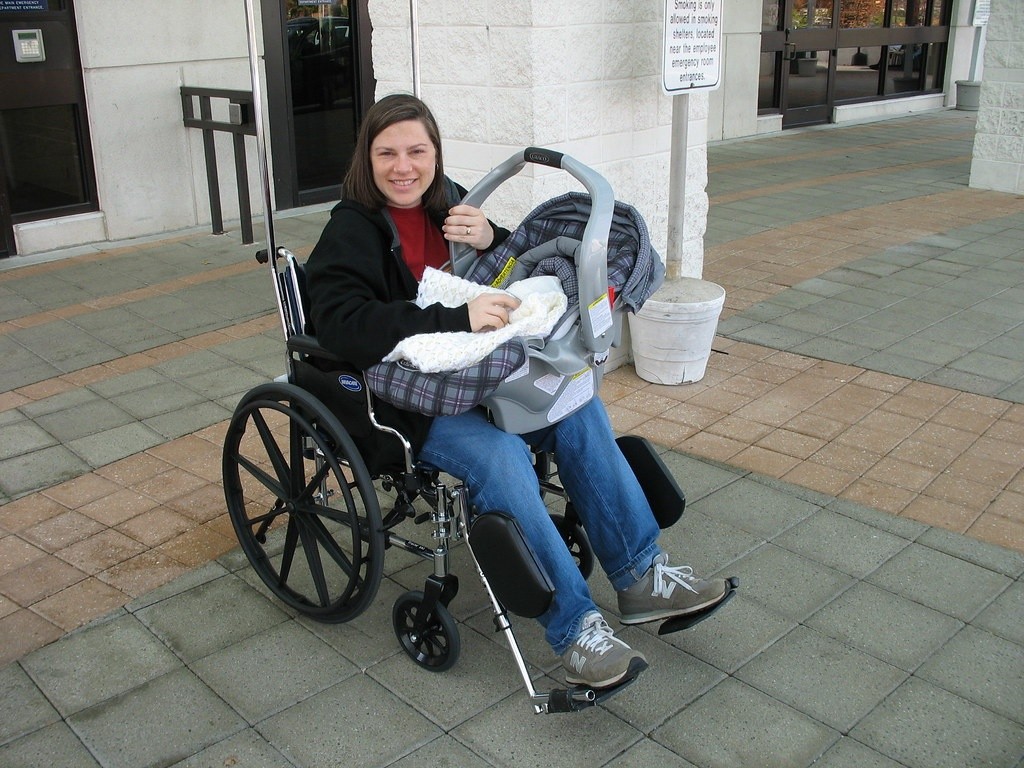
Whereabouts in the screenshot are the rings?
[466,227,472,234]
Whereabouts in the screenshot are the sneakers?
[617,554,731,626]
[561,612,649,691]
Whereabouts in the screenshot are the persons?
[303,95,732,690]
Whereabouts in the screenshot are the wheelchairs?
[220,247,743,717]
